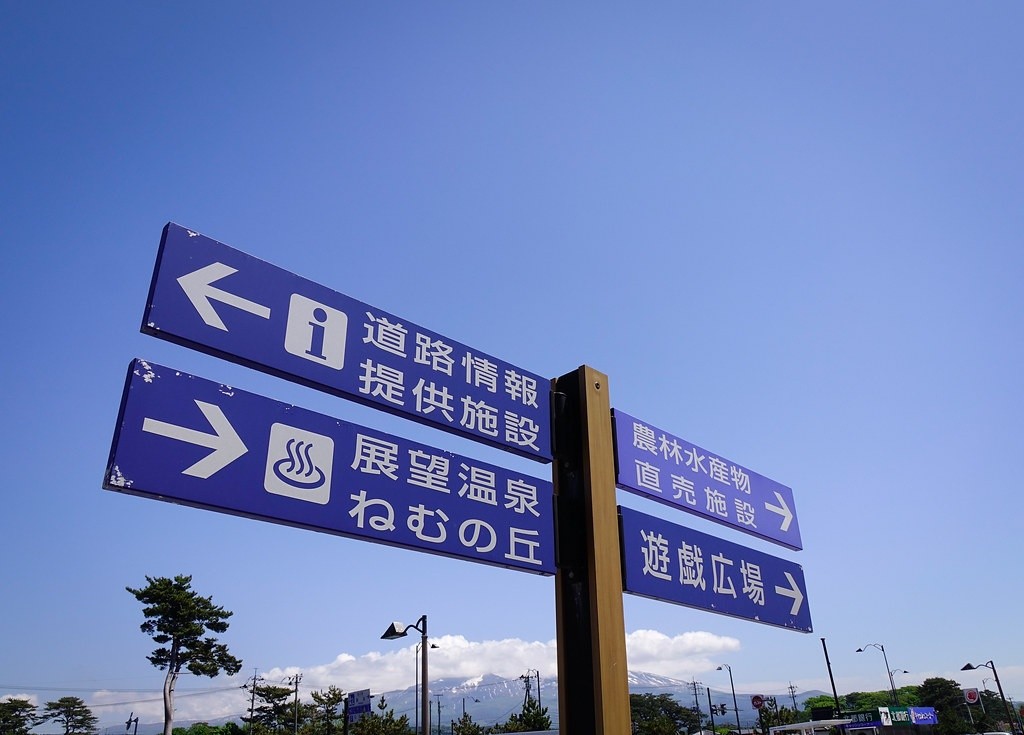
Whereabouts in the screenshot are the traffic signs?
[139,221,555,466]
[102,357,557,578]
[609,406,804,553]
[616,504,813,634]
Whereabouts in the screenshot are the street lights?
[125,712,139,735]
[380,615,429,735]
[463,695,481,717]
[960,660,1017,735]
[716,663,742,735]
[416,641,440,735]
[856,643,909,707]
